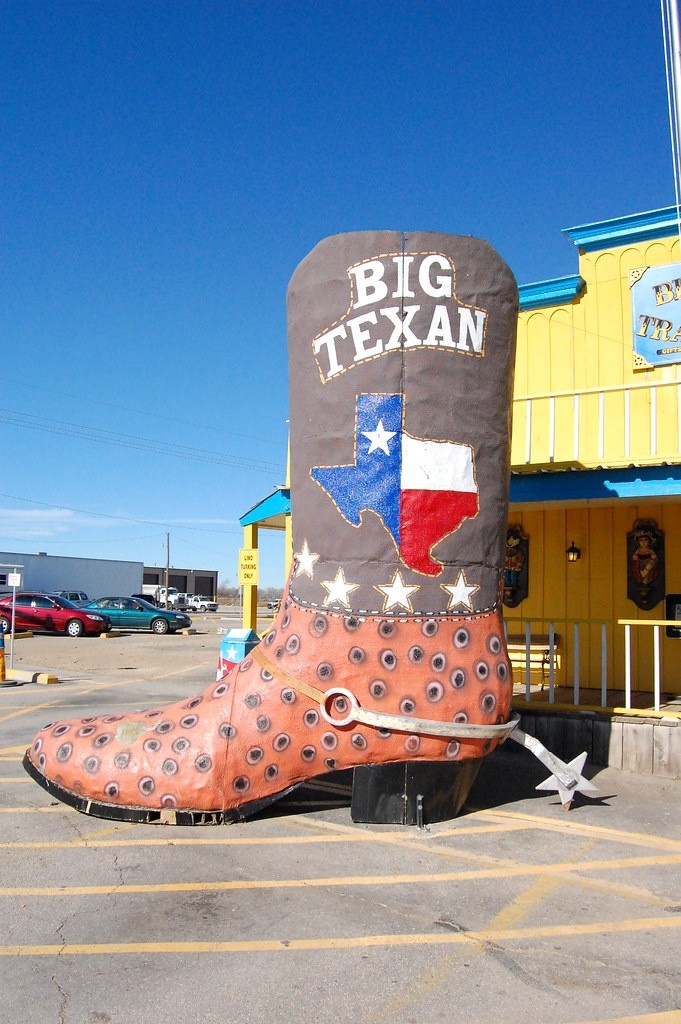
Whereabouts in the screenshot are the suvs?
[266,599,281,609]
[51,590,89,608]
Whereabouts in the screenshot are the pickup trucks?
[176,592,220,613]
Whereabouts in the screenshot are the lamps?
[565,541,581,563]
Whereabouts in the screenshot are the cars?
[80,596,193,635]
[0,592,112,637]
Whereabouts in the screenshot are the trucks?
[141,584,190,611]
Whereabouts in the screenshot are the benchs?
[507,633,560,693]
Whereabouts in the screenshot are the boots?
[21,230,600,824]
